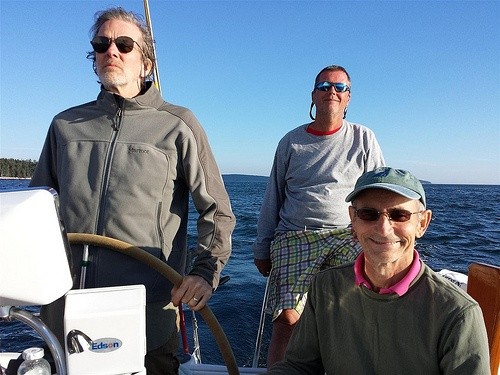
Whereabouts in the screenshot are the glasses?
[315,81,350,93]
[89,35,144,54]
[353,206,424,222]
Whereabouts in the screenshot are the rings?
[193,297,199,303]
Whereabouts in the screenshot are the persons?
[267,167,491,375]
[29,8,236,375]
[253,66,385,372]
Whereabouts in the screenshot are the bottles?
[17,347,51,375]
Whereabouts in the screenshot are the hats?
[345,166,426,209]
